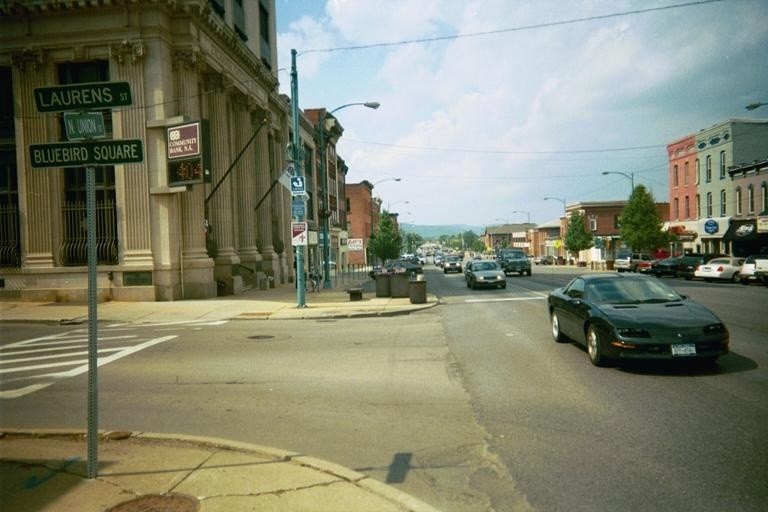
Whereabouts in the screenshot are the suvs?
[495,249,531,276]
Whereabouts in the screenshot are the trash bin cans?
[408,281,427,304]
[376,274,409,298]
[569,260,573,265]
[607,261,613,270]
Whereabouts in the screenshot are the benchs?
[347,287,363,301]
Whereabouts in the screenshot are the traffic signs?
[63,112,106,140]
[28,139,144,168]
[33,82,133,113]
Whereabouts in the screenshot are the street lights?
[545,197,567,217]
[368,178,412,267]
[320,101,380,280]
[602,171,635,195]
[493,211,531,255]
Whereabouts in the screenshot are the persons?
[311,268,319,292]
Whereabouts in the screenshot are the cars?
[613,250,768,284]
[464,255,506,290]
[369,254,423,278]
[435,249,462,273]
[547,276,729,373]
